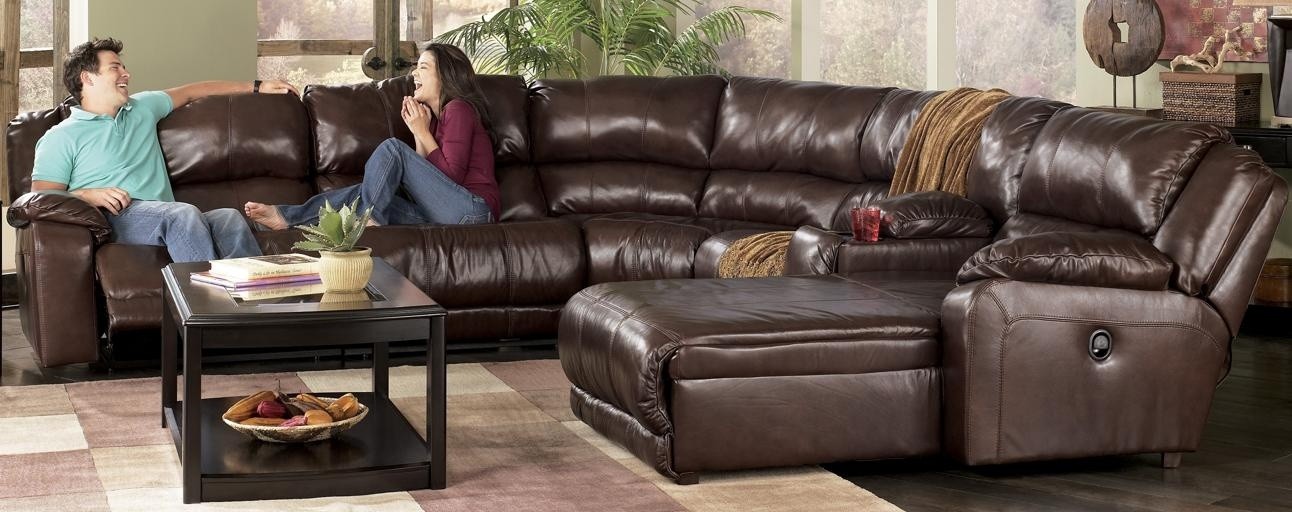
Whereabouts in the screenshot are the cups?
[849,206,881,243]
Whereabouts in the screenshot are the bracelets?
[254,79,263,92]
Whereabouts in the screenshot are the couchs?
[6,75,1289,483]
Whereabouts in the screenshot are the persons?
[31,37,300,263]
[245,43,502,231]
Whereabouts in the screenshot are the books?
[189,253,327,301]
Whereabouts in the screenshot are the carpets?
[0,301,907,510]
